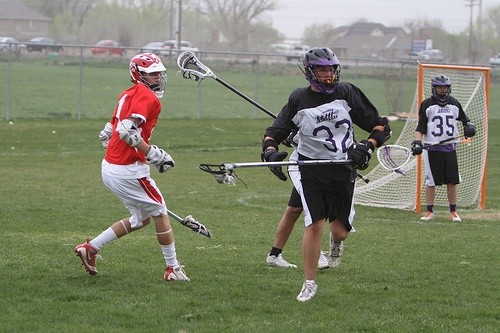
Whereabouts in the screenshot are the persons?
[74,53,191,282]
[260,47,393,302]
[409,73,478,222]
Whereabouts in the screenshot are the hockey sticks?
[178,53,370,184]
[377,134,465,171]
[199,159,354,184]
[166,208,211,238]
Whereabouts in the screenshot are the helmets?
[129,53,167,99]
[431,75,452,105]
[303,47,341,94]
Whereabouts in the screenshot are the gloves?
[412,141,423,156]
[350,139,374,170]
[146,144,175,173]
[464,122,476,138]
[261,150,288,181]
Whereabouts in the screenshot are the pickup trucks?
[285,45,311,62]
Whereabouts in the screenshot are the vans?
[160,40,199,58]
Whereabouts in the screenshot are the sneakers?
[164,265,190,281]
[265,252,297,268]
[74,240,99,275]
[296,282,318,302]
[316,250,330,270]
[451,211,461,222]
[421,211,434,220]
[329,232,344,267]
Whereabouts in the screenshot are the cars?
[137,42,163,56]
[0,37,26,54]
[399,52,429,65]
[91,40,126,56]
[24,37,63,54]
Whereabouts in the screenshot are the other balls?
[52,131,56,133]
[9,122,12,125]
[12,147,15,149]
